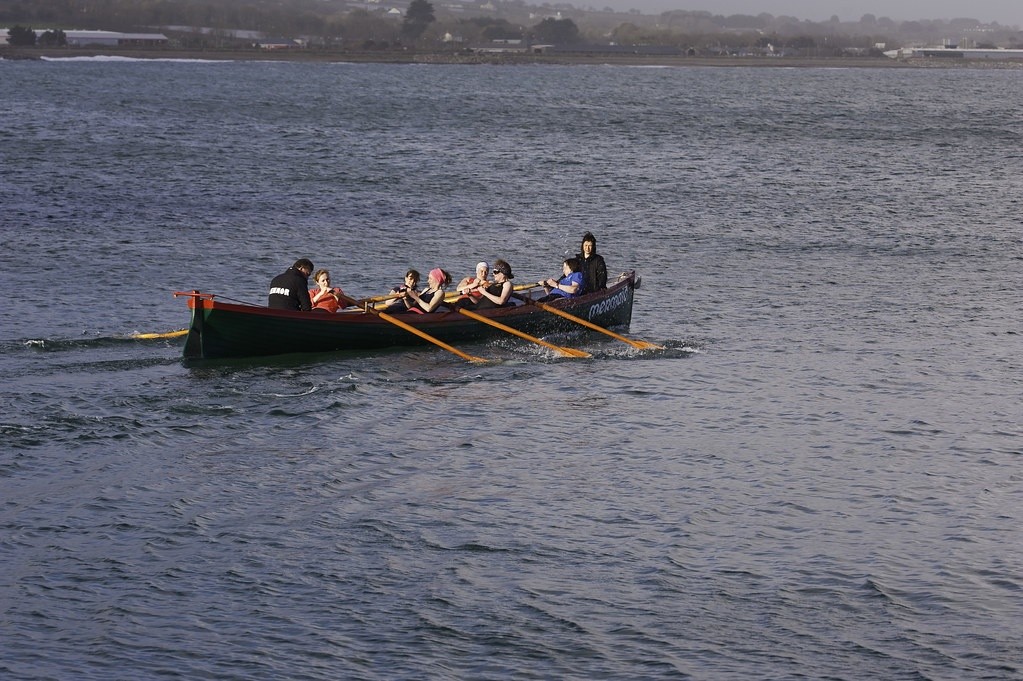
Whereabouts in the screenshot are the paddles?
[130,292,410,339]
[351,288,478,312]
[511,293,659,349]
[513,281,547,291]
[330,290,487,362]
[442,301,590,357]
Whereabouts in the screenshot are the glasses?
[493,270,500,274]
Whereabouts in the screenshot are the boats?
[173,270,641,361]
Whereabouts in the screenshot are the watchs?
[556,283,559,289]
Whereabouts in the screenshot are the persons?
[308,269,347,313]
[536,258,584,303]
[268,259,314,311]
[455,259,513,311]
[575,232,607,295]
[382,268,452,314]
